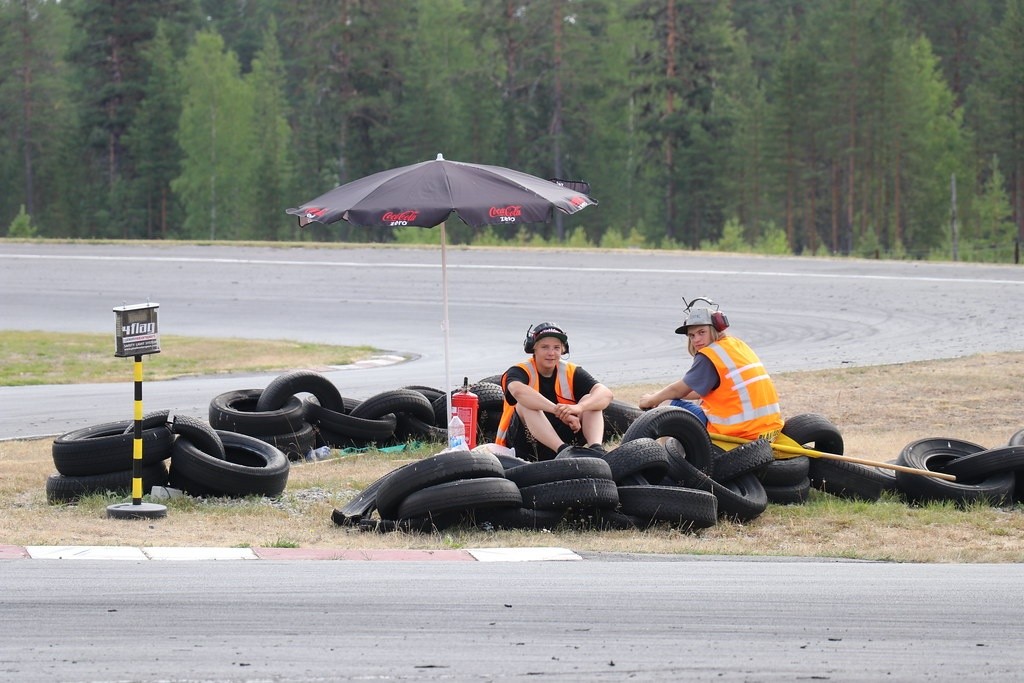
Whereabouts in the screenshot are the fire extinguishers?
[451,376,478,452]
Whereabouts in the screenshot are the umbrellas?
[285,153,598,449]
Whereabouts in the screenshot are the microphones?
[526,324,533,337]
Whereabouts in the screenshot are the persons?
[640,297,785,445]
[494,321,612,461]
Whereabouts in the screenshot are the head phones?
[523,323,570,355]
[682,297,730,332]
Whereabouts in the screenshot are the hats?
[533,328,568,344]
[675,307,714,334]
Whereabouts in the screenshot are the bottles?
[448,413,466,451]
